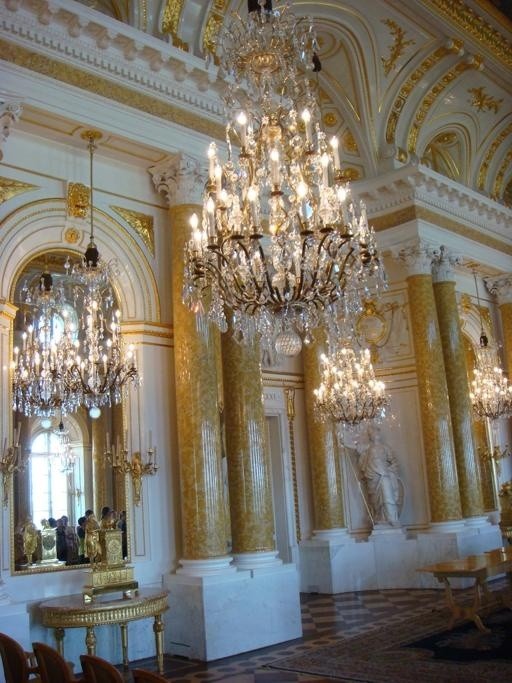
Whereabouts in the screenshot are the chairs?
[30,642,84,681]
[131,667,172,682]
[0,630,74,681]
[79,652,127,682]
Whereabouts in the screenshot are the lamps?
[102,428,111,462]
[474,426,511,475]
[111,425,160,505]
[464,262,512,422]
[311,316,394,427]
[57,117,144,414]
[177,1,394,365]
[50,409,82,476]
[9,253,70,417]
[0,419,31,504]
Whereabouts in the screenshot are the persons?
[45,507,126,564]
[342,426,406,525]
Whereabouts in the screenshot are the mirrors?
[10,247,134,576]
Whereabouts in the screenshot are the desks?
[38,586,170,674]
[412,545,511,634]
